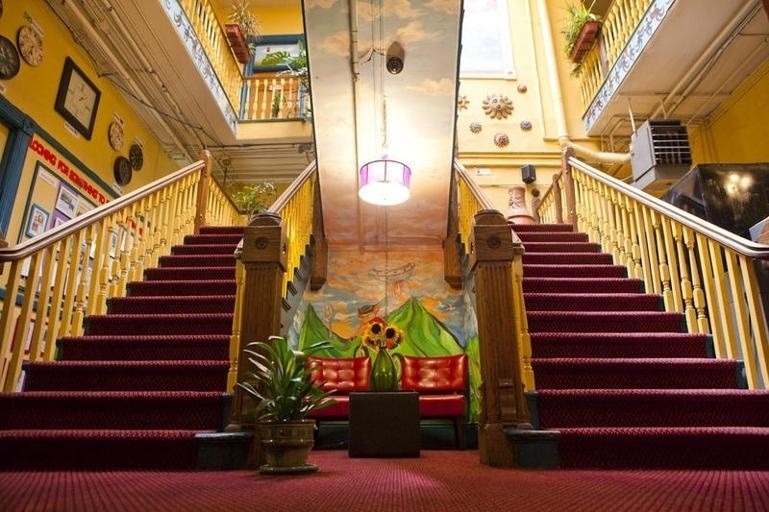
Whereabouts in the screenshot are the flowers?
[365,321,405,348]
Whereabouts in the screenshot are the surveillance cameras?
[387,57,403,74]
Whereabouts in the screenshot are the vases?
[368,349,398,392]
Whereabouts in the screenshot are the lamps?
[357,155,412,207]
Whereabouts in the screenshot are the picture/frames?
[239,34,310,121]
[11,160,142,314]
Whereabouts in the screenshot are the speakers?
[521,164,536,183]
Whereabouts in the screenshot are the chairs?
[306,354,468,450]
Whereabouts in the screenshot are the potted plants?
[561,1,603,80]
[225,0,264,64]
[233,334,340,477]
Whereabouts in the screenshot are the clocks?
[108,122,143,186]
[53,56,101,141]
[1,25,43,80]
